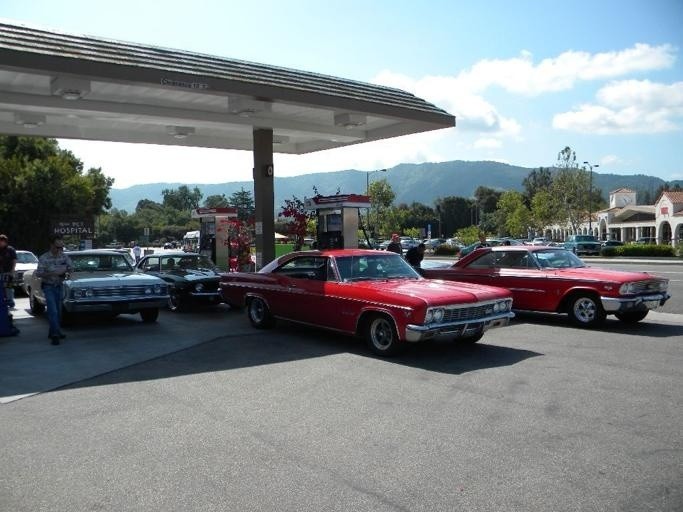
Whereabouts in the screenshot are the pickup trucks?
[104,242,121,249]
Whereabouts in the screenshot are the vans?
[303,238,313,245]
[637,237,655,244]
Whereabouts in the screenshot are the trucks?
[182,230,199,253]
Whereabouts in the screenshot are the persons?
[35,235,71,344]
[359,257,386,277]
[474,233,490,249]
[167,258,175,269]
[405,243,426,267]
[0,233,17,307]
[499,239,512,265]
[386,233,402,256]
[133,244,141,263]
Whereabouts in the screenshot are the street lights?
[584,161,599,234]
[365,168,387,235]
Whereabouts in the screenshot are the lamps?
[335,113,366,129]
[15,113,45,128]
[168,125,193,139]
[273,135,289,146]
[231,97,272,118]
[51,76,90,100]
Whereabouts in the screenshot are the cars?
[419,245,668,325]
[152,239,180,249]
[359,235,624,260]
[12,249,226,327]
[217,249,514,356]
